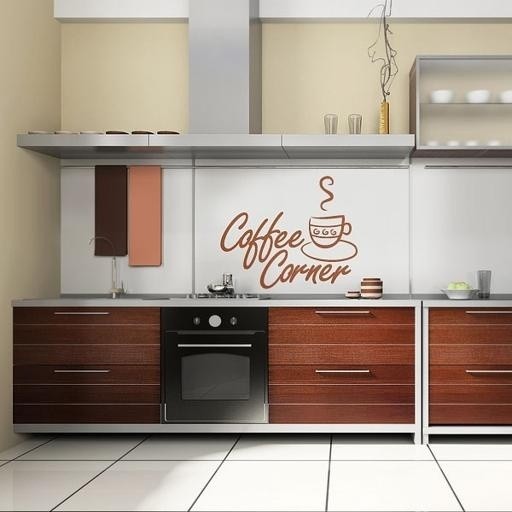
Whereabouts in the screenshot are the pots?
[206,284,234,294]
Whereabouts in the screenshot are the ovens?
[161,329,269,423]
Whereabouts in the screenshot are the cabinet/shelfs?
[409,55,512,158]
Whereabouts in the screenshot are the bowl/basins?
[424,90,511,102]
[441,289,479,300]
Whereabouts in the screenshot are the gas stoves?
[160,293,270,331]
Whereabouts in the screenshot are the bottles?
[223,274,232,287]
[361,277,382,299]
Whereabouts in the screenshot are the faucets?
[89,237,116,298]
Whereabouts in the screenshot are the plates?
[346,295,360,298]
[26,130,180,135]
[427,141,499,146]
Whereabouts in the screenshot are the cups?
[348,291,360,296]
[307,215,352,248]
[324,114,362,134]
[477,271,491,298]
